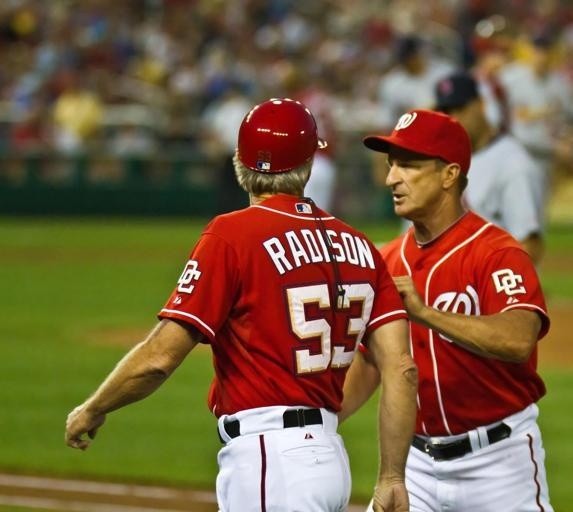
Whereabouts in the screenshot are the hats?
[434,75,477,110]
[238,98,328,173]
[362,109,471,177]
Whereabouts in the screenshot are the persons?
[433,71,547,263]
[337,109,554,511]
[65,96,419,512]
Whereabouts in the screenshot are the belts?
[411,423,511,459]
[217,408,323,443]
